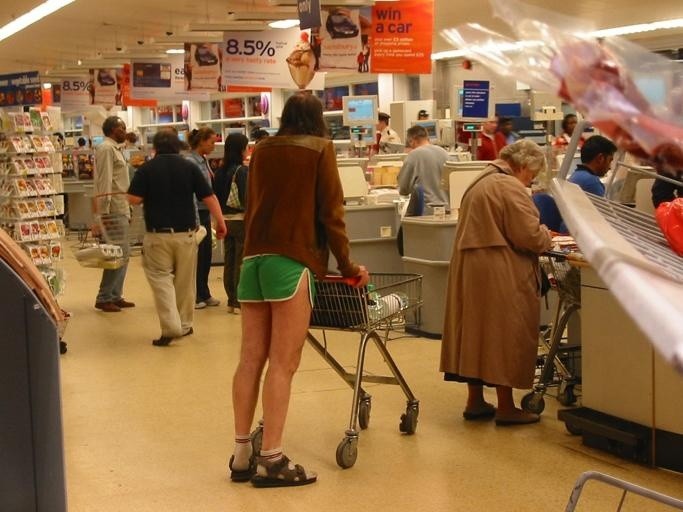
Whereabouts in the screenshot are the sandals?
[251,453,317,488]
[229,454,256,482]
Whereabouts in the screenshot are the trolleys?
[251,271,424,464]
[518,242,581,416]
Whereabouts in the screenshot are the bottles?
[367,284,382,321]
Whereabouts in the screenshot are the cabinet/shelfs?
[580,262,683,475]
[184,87,283,148]
[125,99,190,137]
[0,107,64,297]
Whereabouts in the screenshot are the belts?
[145,224,200,234]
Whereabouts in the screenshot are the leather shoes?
[462,400,496,417]
[153,336,173,346]
[181,327,194,336]
[94,300,121,313]
[112,298,135,308]
[492,407,542,424]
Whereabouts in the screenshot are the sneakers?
[195,302,207,309]
[204,296,221,306]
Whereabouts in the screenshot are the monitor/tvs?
[342,94,378,126]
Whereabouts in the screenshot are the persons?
[184,126,222,310]
[212,131,250,315]
[498,118,514,145]
[547,134,618,393]
[357,50,364,72]
[362,44,370,73]
[255,129,269,144]
[93,114,136,314]
[417,109,430,121]
[468,115,508,161]
[554,113,586,146]
[378,111,400,154]
[125,126,229,347]
[53,131,64,145]
[436,138,552,423]
[123,131,139,161]
[227,89,371,488]
[77,136,88,151]
[395,125,454,217]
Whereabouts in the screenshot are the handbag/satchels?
[226,180,245,211]
[541,266,551,297]
